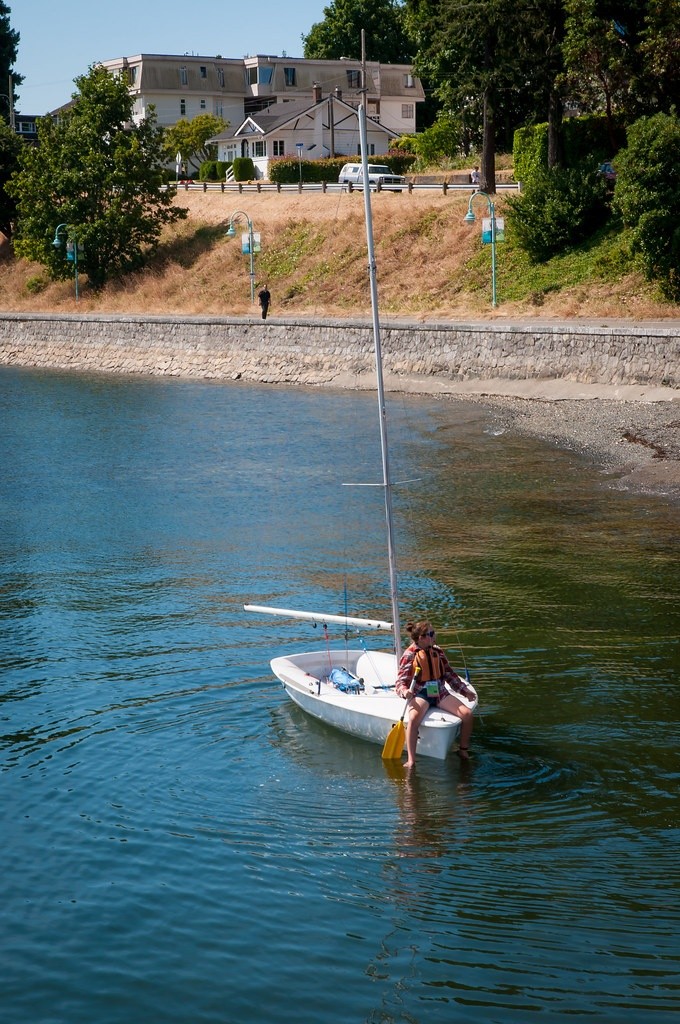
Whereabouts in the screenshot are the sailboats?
[240,26,479,759]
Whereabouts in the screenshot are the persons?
[471,166,481,194]
[258,284,271,319]
[395,621,477,767]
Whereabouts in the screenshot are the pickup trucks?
[338,162,403,193]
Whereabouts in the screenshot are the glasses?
[422,631,435,637]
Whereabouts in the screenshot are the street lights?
[226,211,256,303]
[53,223,80,304]
[461,192,497,309]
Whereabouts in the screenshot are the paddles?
[381,666,421,759]
